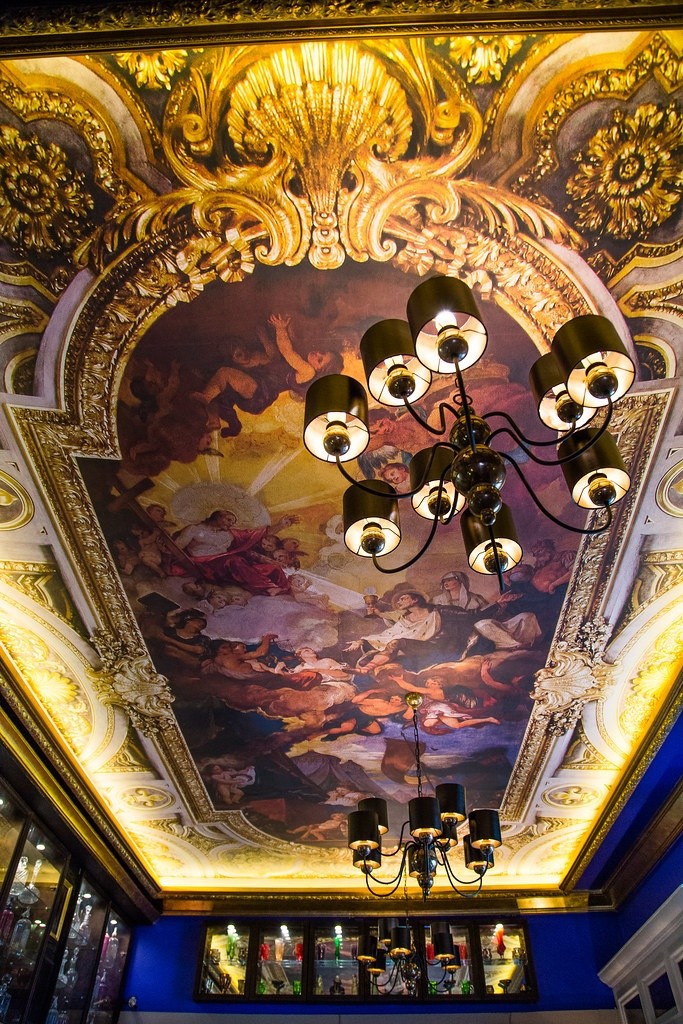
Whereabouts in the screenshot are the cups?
[317,937,358,964]
[426,944,529,995]
[275,938,283,963]
[293,979,301,995]
[209,935,247,965]
[220,974,231,988]
[256,981,265,994]
[258,944,271,960]
[202,978,214,991]
[238,980,245,994]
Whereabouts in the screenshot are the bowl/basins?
[295,944,303,960]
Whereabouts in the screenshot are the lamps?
[36,838,45,850]
[303,276,634,593]
[348,700,500,910]
[354,861,462,1000]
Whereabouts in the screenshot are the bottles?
[496,932,506,955]
[0,856,125,1024]
[371,976,379,995]
[313,974,358,995]
